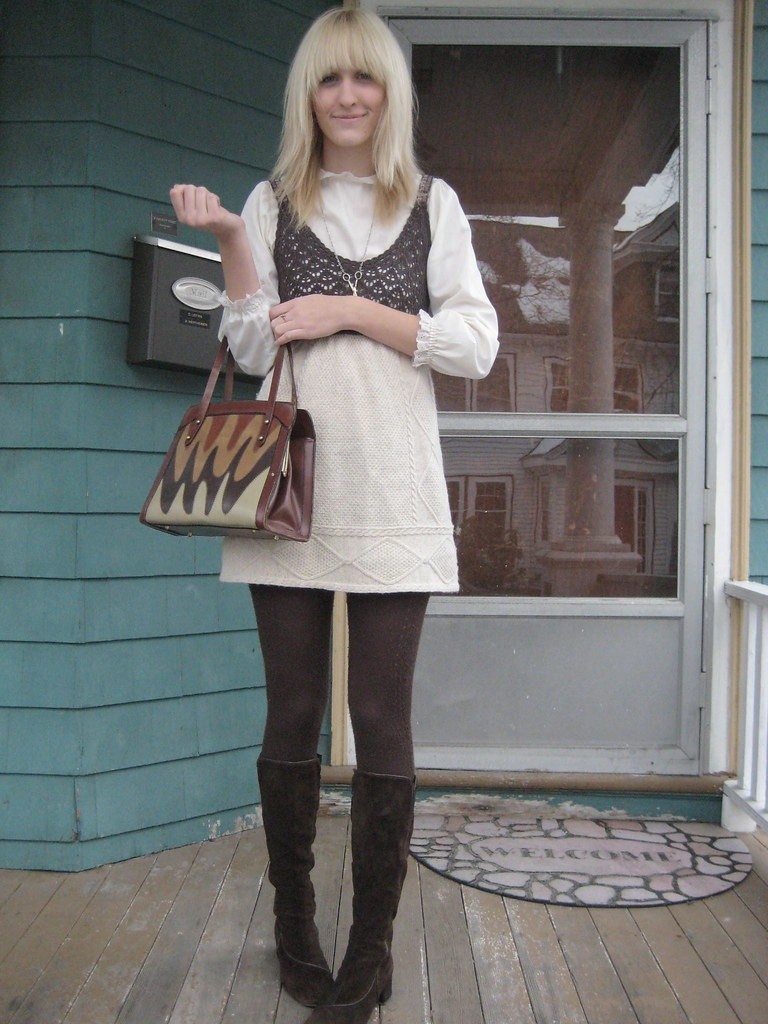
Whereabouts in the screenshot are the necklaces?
[318,192,376,296]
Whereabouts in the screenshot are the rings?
[280,314,287,323]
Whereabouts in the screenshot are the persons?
[169,6,500,1023]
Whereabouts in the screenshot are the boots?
[257,749,335,1003]
[301,768,417,1024]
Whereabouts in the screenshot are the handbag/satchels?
[138,334,316,544]
